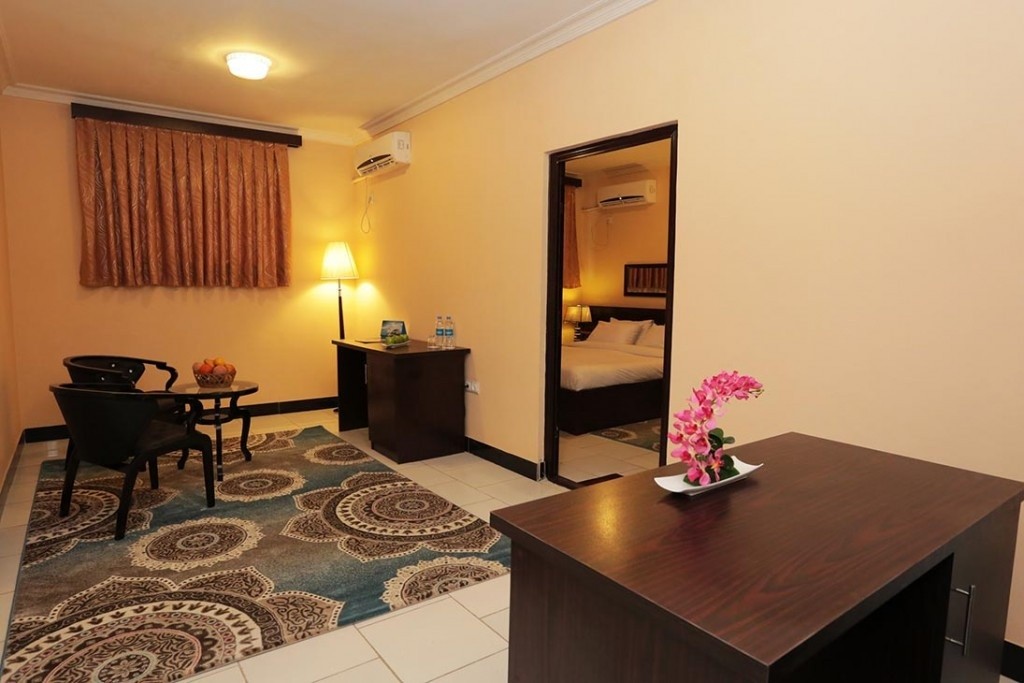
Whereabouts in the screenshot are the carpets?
[0,426,512,683]
[593,418,661,453]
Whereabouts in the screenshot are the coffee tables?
[168,380,259,481]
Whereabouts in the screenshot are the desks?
[490,432,1024,683]
[332,338,471,465]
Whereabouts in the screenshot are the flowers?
[669,370,764,485]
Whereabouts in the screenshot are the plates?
[381,340,412,349]
[654,455,764,497]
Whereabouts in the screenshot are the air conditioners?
[596,180,656,209]
[354,131,411,176]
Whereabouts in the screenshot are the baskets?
[193,370,236,388]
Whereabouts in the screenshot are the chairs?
[48,355,215,541]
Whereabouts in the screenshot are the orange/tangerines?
[198,364,213,375]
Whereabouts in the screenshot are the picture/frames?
[624,264,667,297]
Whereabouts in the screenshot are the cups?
[427,334,435,346]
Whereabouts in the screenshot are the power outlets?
[465,381,479,393]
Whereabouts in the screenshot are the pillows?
[588,317,665,347]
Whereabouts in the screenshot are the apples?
[385,333,408,344]
[192,357,234,375]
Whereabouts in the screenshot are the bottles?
[386,332,408,344]
[434,316,444,347]
[444,316,455,349]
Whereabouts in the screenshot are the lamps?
[566,305,592,341]
[323,241,357,339]
[226,52,273,81]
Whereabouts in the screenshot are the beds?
[557,317,666,436]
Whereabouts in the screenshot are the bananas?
[204,359,215,368]
